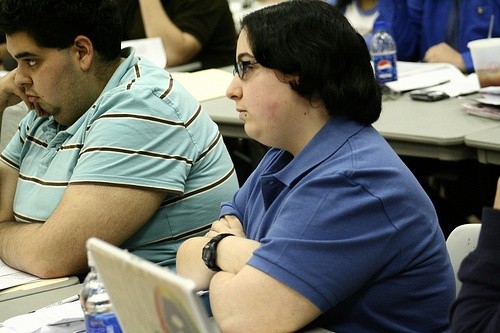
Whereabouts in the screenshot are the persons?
[0,0,240,280]
[175,0,456,333]
[0,0,500,77]
[447,175,500,333]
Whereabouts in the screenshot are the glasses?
[233,59,259,79]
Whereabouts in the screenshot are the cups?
[467,37,500,89]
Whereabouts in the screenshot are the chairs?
[444,222,482,298]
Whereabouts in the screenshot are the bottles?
[370,21,401,100]
[78,250,122,333]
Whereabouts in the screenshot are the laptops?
[87,237,334,333]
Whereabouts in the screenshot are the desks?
[0,276,81,301]
[463,121,500,165]
[165,61,203,75]
[370,91,476,162]
[0,283,84,323]
[199,63,256,165]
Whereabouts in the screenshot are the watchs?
[202,233,235,272]
